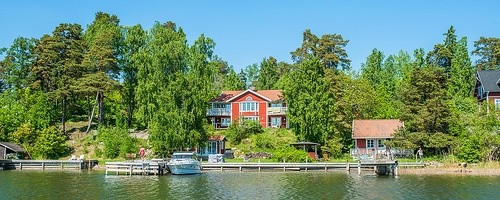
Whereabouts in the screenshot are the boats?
[166,151,203,175]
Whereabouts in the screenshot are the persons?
[416,147,423,162]
[139,146,145,160]
